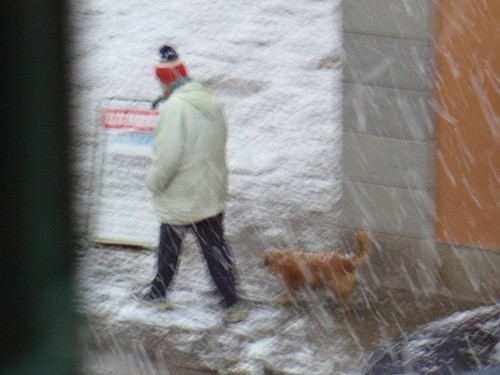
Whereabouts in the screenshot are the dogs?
[264,231,372,308]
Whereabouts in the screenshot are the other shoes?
[224,303,250,323]
[131,290,167,307]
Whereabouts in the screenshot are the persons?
[129,46,249,322]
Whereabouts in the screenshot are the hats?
[154,44,188,86]
